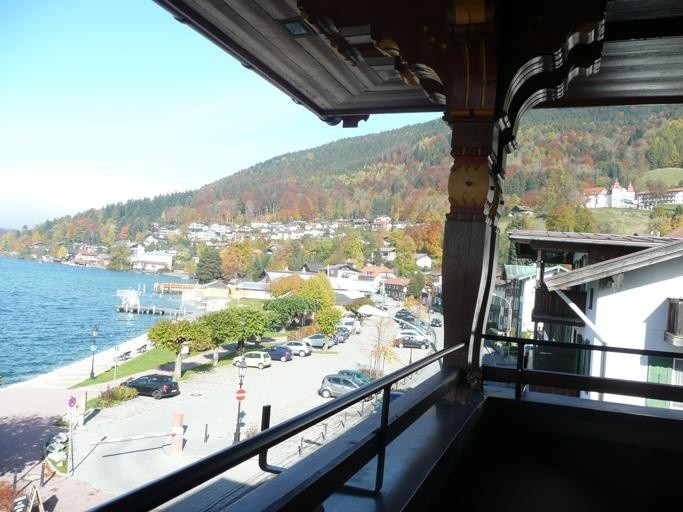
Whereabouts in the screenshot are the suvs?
[233,351,271,369]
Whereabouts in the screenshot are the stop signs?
[235,388,247,401]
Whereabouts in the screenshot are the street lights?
[491,292,511,364]
[357,303,445,369]
[88,324,98,379]
[231,356,248,444]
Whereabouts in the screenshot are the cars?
[303,334,335,348]
[339,369,380,393]
[327,318,361,344]
[259,345,291,361]
[120,374,180,400]
[319,375,372,403]
[431,318,442,327]
[394,308,431,349]
[376,303,388,310]
[279,340,312,357]
